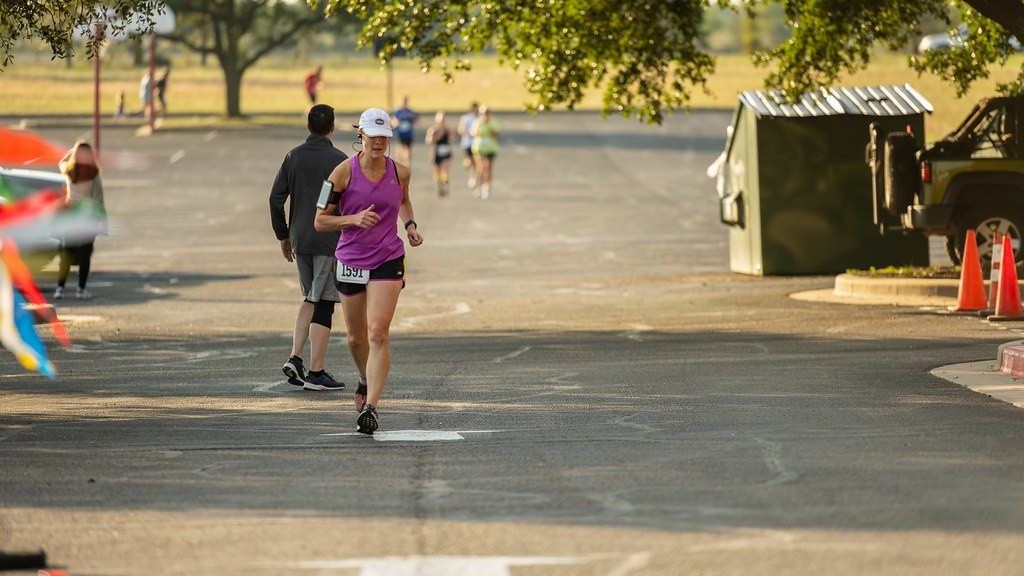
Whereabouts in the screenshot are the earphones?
[357,134,363,145]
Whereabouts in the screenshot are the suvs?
[866,94,1024,281]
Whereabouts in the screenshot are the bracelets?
[404,220,417,230]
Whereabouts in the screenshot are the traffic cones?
[978,231,1004,316]
[987,236,1024,321]
[949,228,990,315]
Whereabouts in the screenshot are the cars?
[919,21,1022,52]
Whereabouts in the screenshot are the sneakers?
[303,372,346,391]
[356,404,378,435]
[355,381,367,412]
[282,356,307,386]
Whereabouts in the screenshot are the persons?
[113,66,169,115]
[268,103,350,391]
[305,66,322,103]
[425,110,456,196]
[54,141,109,300]
[314,107,423,436]
[470,105,502,199]
[458,103,482,189]
[391,97,420,168]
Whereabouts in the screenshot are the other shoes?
[54,287,64,298]
[76,290,92,299]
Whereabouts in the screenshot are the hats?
[358,108,393,138]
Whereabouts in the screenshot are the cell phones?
[316,180,332,210]
